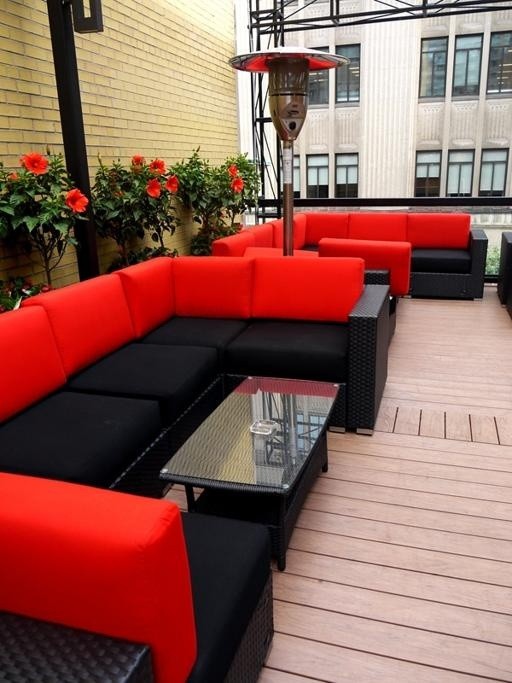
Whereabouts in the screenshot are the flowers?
[0,274,55,313]
[129,154,179,251]
[0,146,91,290]
[220,153,261,234]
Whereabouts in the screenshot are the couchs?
[213,211,487,300]
[1,254,392,683]
